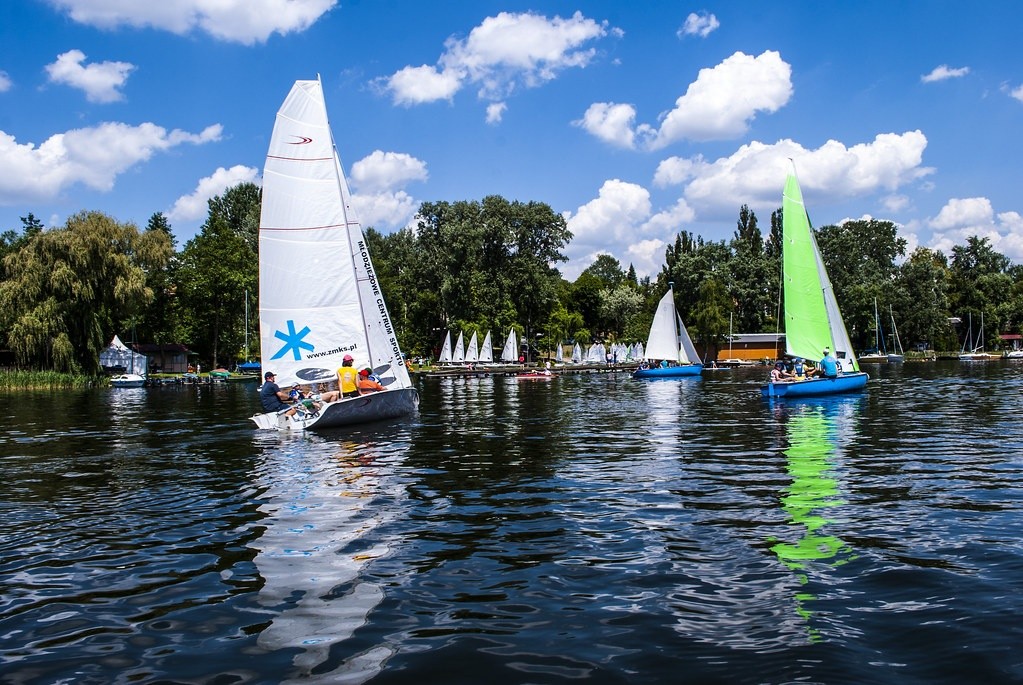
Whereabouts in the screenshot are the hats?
[823,349,829,354]
[775,362,783,368]
[265,372,277,380]
[795,358,802,363]
[358,369,368,376]
[342,354,354,362]
[641,361,645,364]
[365,368,372,375]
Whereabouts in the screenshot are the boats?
[209,368,232,383]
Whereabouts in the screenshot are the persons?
[638,358,667,370]
[770,357,818,383]
[365,367,382,386]
[710,361,717,368]
[819,349,838,378]
[260,372,296,417]
[358,370,387,395]
[406,356,423,372]
[607,351,613,367]
[467,363,476,371]
[188,376,201,384]
[197,363,200,374]
[289,381,338,414]
[519,355,524,370]
[337,354,363,399]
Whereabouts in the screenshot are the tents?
[99,334,147,376]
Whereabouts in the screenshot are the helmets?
[291,382,300,389]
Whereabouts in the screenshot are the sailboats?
[957,311,1023,360]
[109,316,146,388]
[633,282,704,377]
[248,72,421,431]
[721,311,756,368]
[237,289,261,374]
[437,326,645,373]
[760,157,870,397]
[858,296,906,363]
